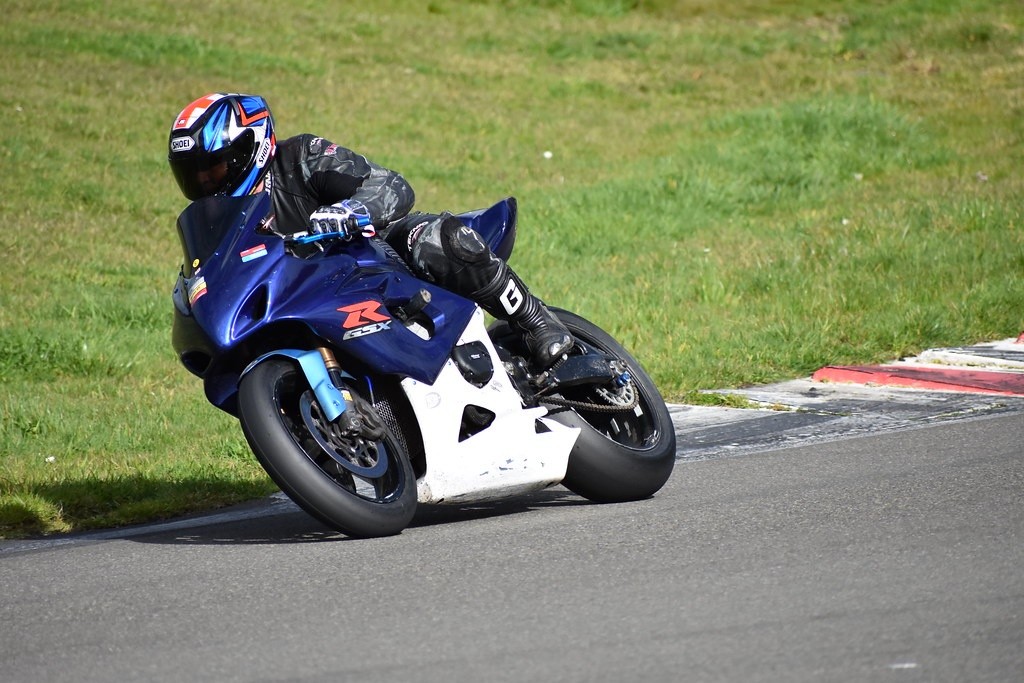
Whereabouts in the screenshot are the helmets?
[168,92,278,201]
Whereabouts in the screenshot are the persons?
[167,92,575,380]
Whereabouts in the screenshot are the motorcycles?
[170,192,678,538]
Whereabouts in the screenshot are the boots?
[468,258,573,369]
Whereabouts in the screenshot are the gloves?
[309,199,371,252]
